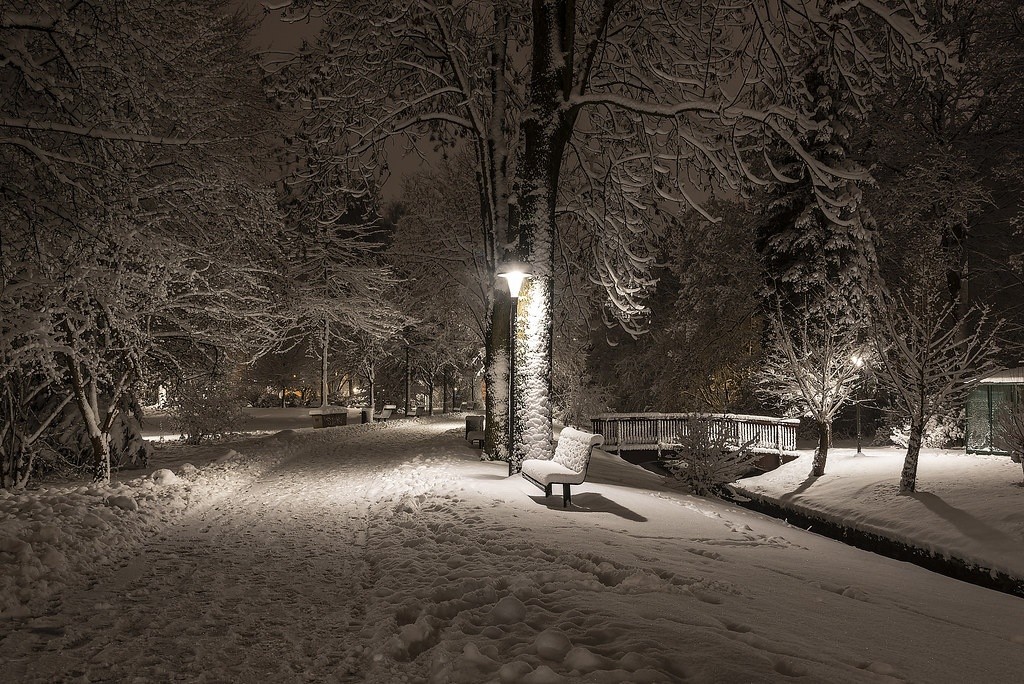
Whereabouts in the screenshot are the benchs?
[521,426,604,509]
[407,407,424,417]
[373,405,396,422]
[467,429,485,448]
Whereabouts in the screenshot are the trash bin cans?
[466,416,484,440]
[362,408,373,424]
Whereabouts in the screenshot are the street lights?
[499,268,532,455]
[851,350,875,453]
